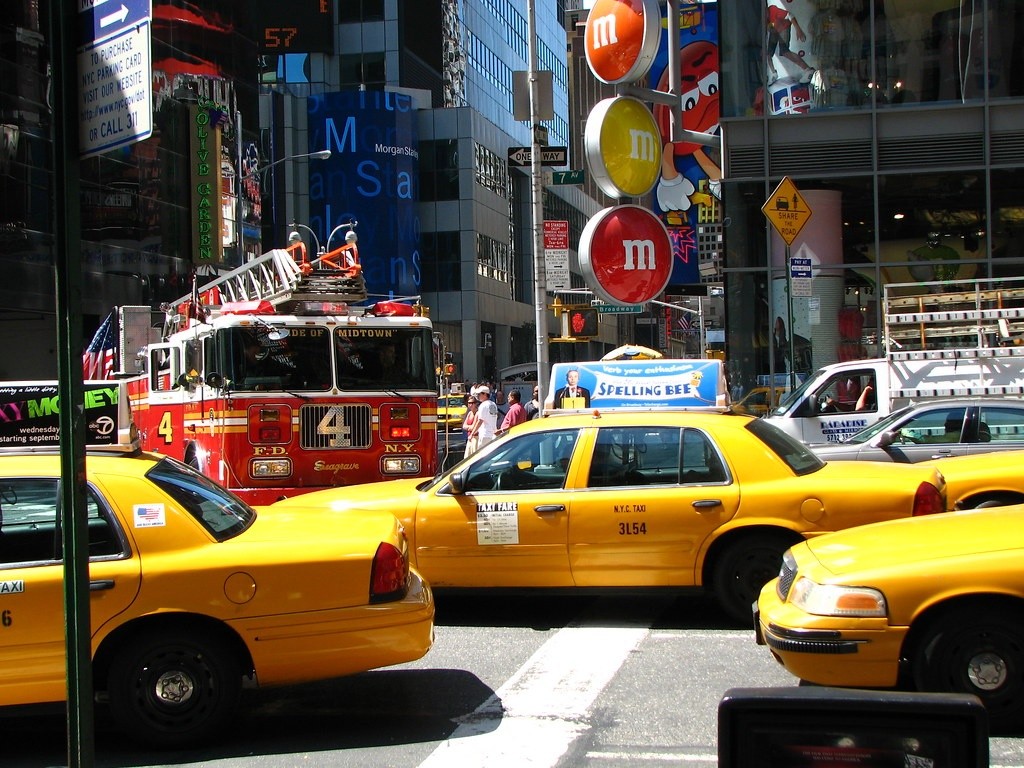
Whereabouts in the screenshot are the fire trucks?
[125,300,454,506]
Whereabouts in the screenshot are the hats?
[468,399,475,402]
[474,386,489,394]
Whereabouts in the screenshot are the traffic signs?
[508,123,569,167]
[73,3,153,158]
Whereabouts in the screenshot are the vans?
[759,351,1024,444]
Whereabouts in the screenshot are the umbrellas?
[600,344,663,361]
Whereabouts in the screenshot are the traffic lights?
[568,309,599,337]
[444,364,456,374]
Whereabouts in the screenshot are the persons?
[902,410,964,444]
[826,376,877,411]
[463,379,539,459]
[555,370,590,409]
[371,341,396,377]
[772,317,790,373]
[233,340,267,390]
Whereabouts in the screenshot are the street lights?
[237,111,331,270]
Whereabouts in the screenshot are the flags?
[82,308,120,380]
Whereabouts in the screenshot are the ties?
[571,387,575,393]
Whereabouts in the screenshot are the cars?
[801,397,1024,462]
[751,504,1024,726]
[0,454,439,750]
[922,449,1024,511]
[264,412,948,623]
[726,386,785,416]
[437,392,471,433]
